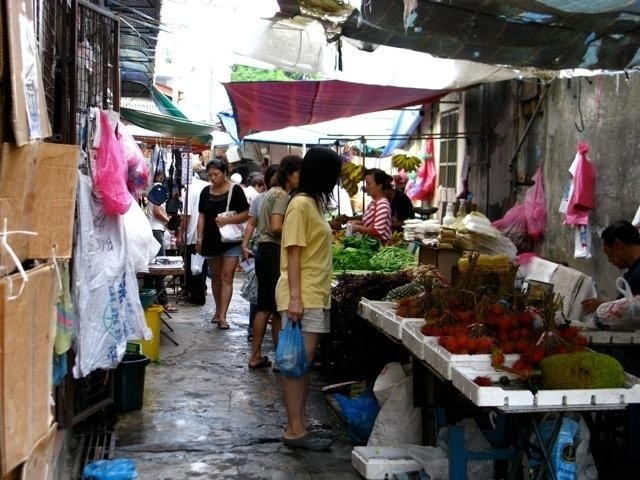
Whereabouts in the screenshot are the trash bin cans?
[139,288,157,309]
[118,353,151,412]
[140,304,165,363]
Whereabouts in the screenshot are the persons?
[146,154,416,341]
[580,218,640,316]
[246,154,301,369]
[195,156,251,330]
[273,144,343,452]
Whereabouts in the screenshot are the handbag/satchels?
[147,177,181,230]
[218,210,246,242]
[232,257,258,304]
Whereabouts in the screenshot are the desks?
[134,254,205,345]
[323,266,640,480]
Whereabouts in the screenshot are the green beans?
[368,244,419,272]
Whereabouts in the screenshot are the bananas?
[392,153,421,173]
[341,162,366,197]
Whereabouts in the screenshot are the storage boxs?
[408,236,518,286]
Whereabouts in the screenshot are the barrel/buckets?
[115,354,150,413]
[138,305,165,364]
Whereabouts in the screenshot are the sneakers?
[211,314,230,329]
[248,356,271,369]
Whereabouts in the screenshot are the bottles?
[455,199,467,224]
[442,202,456,226]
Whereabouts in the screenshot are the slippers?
[283,419,332,451]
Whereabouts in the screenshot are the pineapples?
[388,283,421,300]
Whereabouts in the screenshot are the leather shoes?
[178,292,204,306]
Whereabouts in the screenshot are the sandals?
[163,302,178,312]
